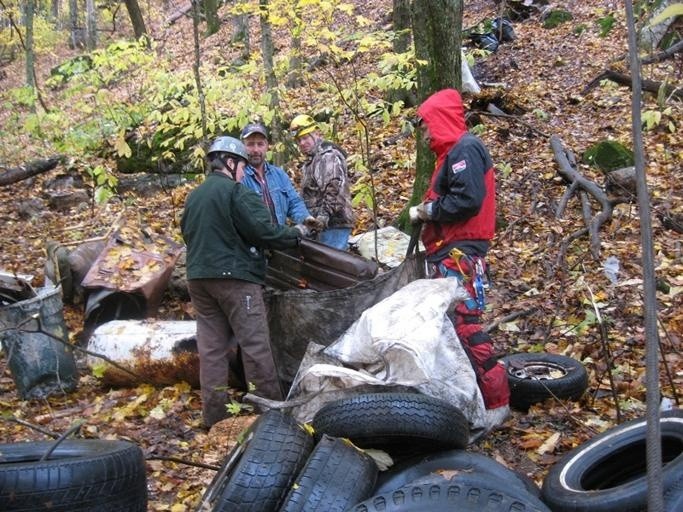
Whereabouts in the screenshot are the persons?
[408,86,513,432]
[288,114,352,248]
[179,135,308,432]
[236,122,325,233]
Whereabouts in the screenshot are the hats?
[242,124,268,139]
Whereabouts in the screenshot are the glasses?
[290,122,316,137]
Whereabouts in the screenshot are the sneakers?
[483,401,509,428]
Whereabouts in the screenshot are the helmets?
[206,136,248,162]
[290,114,321,139]
